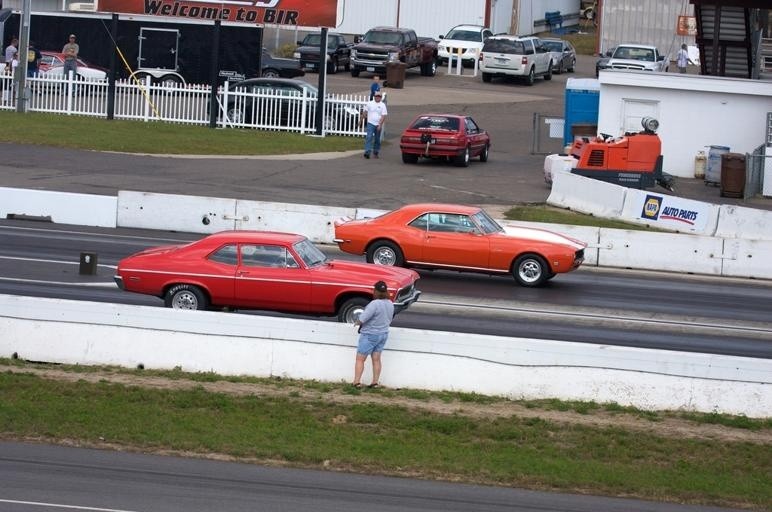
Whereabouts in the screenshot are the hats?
[372,282,387,292]
[375,92,382,96]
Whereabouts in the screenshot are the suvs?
[478,32,552,85]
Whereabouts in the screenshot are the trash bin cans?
[383,61,408,89]
[720,152,745,199]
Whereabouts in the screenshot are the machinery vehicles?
[542,116,677,194]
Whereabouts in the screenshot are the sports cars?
[336,201,586,287]
[114,232,421,325]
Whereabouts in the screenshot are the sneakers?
[364,151,379,159]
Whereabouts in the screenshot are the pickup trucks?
[292,33,354,73]
[607,44,670,72]
[349,26,438,78]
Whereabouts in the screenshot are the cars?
[35,50,109,96]
[262,47,305,86]
[437,24,493,66]
[541,38,577,74]
[208,79,367,135]
[399,114,491,166]
[597,48,615,76]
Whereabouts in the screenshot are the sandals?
[351,383,379,389]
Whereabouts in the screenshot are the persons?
[4,37,43,97]
[348,279,395,390]
[356,91,387,159]
[60,34,80,97]
[675,43,696,72]
[368,75,381,102]
